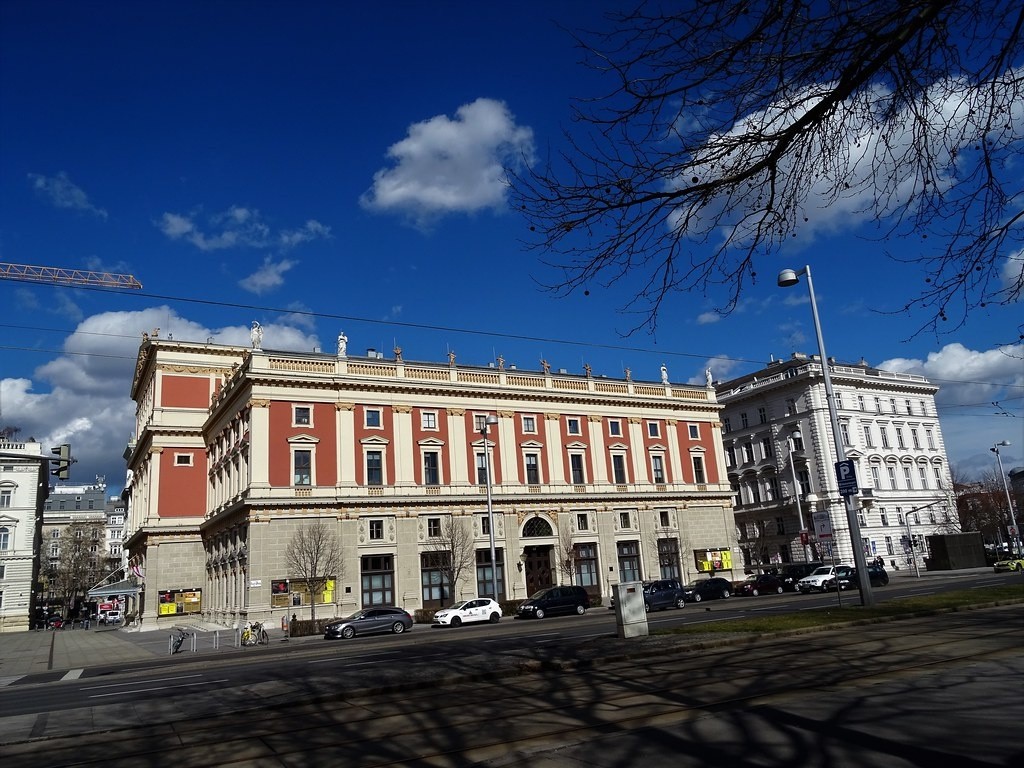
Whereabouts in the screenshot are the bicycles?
[173,628,189,654]
[241,621,269,647]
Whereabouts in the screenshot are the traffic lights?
[50,444,71,481]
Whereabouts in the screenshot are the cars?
[798,565,889,594]
[324,607,413,639]
[516,586,590,619]
[684,578,733,602]
[433,598,502,628]
[994,554,1024,574]
[610,579,687,613]
[735,575,784,597]
[99,609,120,623]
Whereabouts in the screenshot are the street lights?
[786,431,811,564]
[480,415,498,603]
[990,440,1022,554]
[287,580,290,641]
[777,265,875,606]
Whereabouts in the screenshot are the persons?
[873,556,884,568]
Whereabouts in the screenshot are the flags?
[133,566,144,578]
